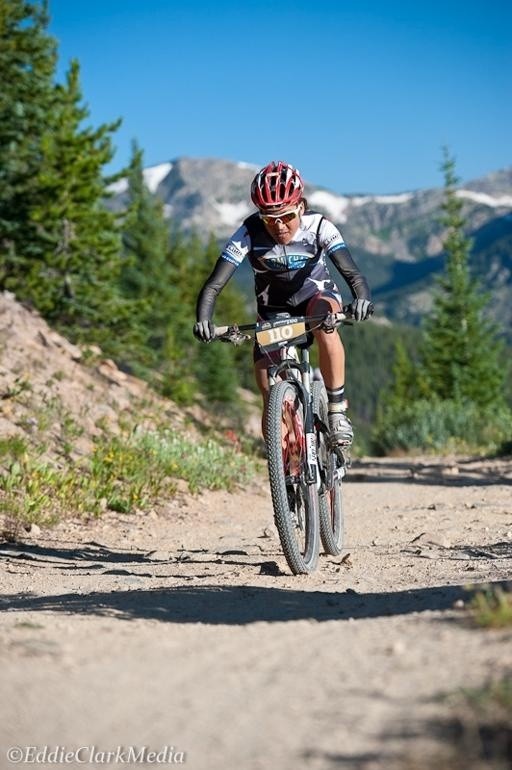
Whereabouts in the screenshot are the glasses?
[257,213,297,224]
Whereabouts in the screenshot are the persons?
[191,161,373,528]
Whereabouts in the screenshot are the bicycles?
[199,301,374,576]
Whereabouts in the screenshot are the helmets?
[250,160,305,216]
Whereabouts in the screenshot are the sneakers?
[327,412,352,449]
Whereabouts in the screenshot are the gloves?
[329,247,374,323]
[193,259,237,344]
